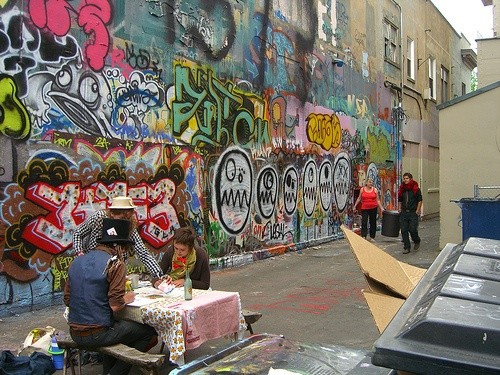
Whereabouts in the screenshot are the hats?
[107,196,137,209]
[95,218,136,244]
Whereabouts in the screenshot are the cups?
[131,274,139,289]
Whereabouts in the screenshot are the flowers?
[173,255,192,271]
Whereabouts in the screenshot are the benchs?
[241,310,262,335]
[56,334,165,375]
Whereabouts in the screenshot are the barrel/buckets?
[381,210,401,237]
[46,337,70,370]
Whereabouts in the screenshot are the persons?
[397,172,423,254]
[72,197,173,285]
[353,177,385,242]
[64,217,158,375]
[151,227,211,290]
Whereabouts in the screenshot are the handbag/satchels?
[22,325,56,356]
[0,348,56,375]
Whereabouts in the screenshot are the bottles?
[184,265,192,300]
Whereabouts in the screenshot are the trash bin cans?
[459,197,500,241]
[381,210,401,237]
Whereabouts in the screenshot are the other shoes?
[414,240,420,249]
[363,237,366,239]
[403,247,410,254]
[370,237,375,242]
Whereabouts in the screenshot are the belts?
[70,326,105,336]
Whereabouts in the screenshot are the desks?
[61,285,247,365]
